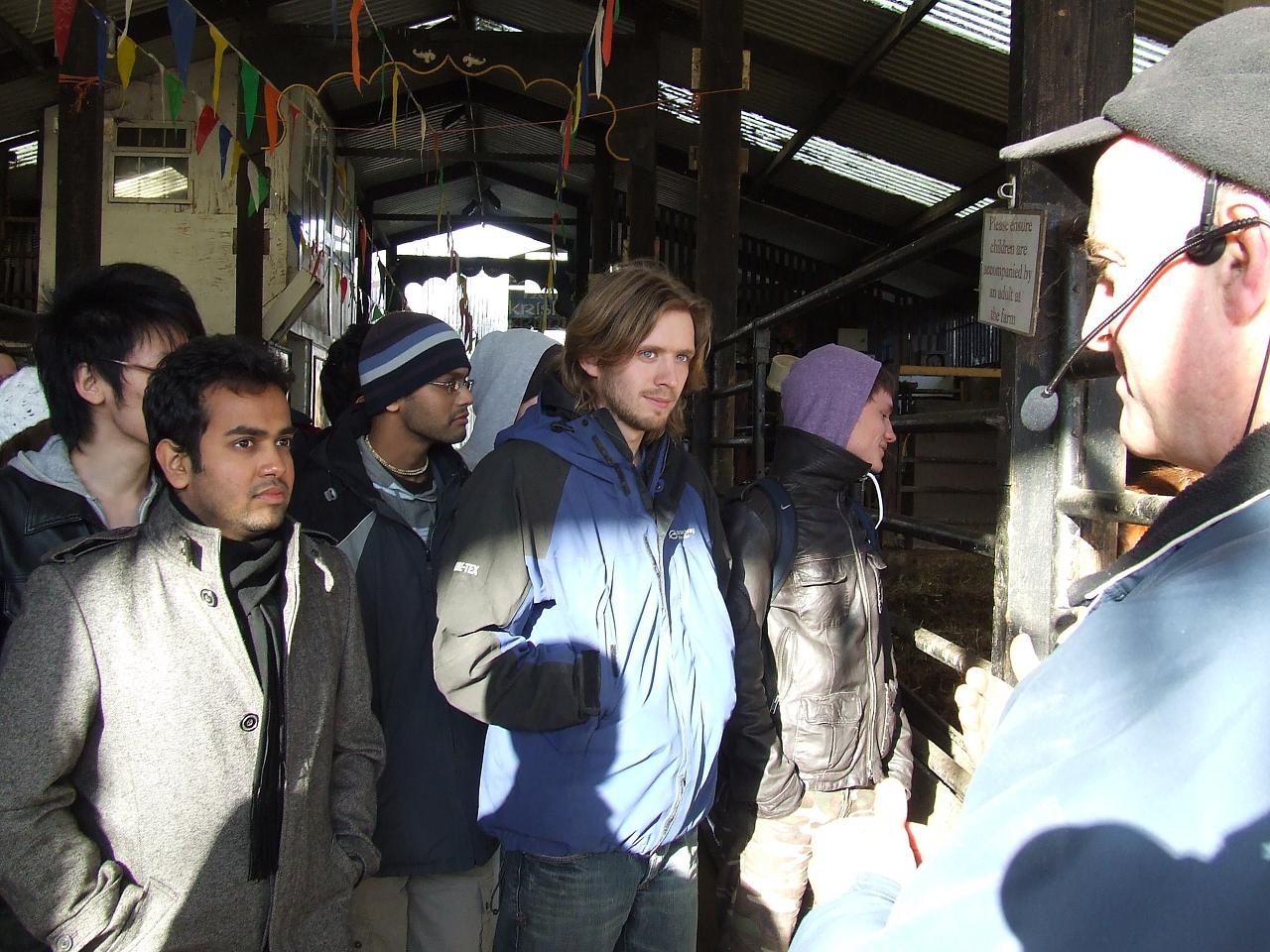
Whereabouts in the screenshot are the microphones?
[1022,217,1263,433]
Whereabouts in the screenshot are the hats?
[358,311,472,418]
[998,5,1270,201]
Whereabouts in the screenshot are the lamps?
[441,105,467,131]
[460,200,478,218]
[482,189,501,211]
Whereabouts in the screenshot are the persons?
[0,262,208,644]
[784,4,1270,952]
[433,262,736,952]
[0,335,387,952]
[457,326,564,472]
[725,340,914,952]
[282,309,487,952]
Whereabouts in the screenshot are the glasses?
[428,379,476,394]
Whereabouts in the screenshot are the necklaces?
[363,436,432,477]
